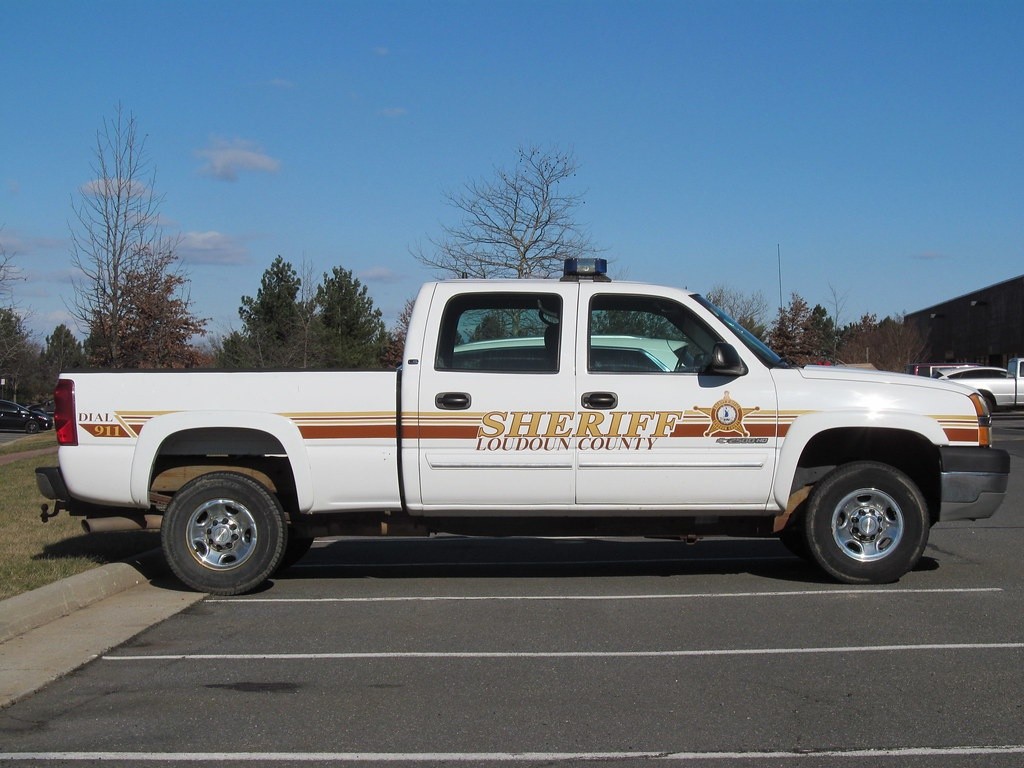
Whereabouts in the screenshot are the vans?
[906,363,984,377]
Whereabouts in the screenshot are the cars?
[31,399,54,413]
[931,367,1007,379]
[0,400,52,433]
[454,335,695,372]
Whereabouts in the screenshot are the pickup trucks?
[945,358,1024,413]
[35,255,1012,596]
